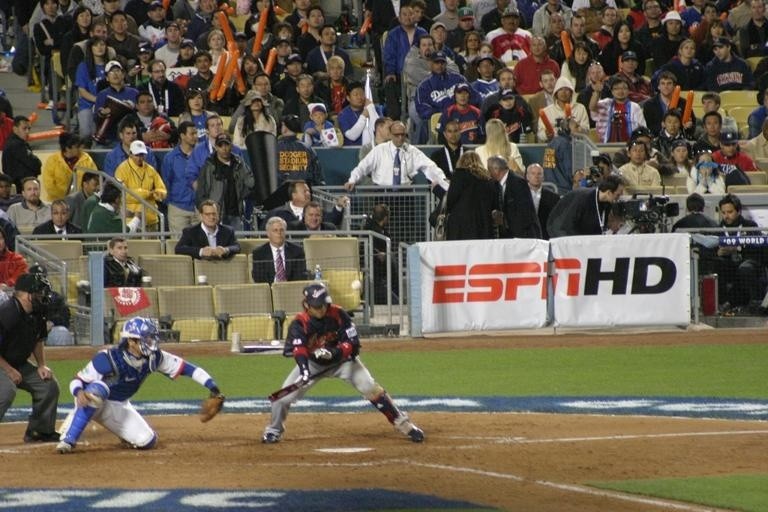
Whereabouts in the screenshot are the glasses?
[725,143,737,146]
[392,133,405,137]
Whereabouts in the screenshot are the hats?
[241,90,270,107]
[303,283,332,306]
[661,10,686,27]
[501,7,519,17]
[621,51,639,62]
[215,133,231,145]
[281,114,300,133]
[454,83,471,93]
[105,60,122,72]
[712,38,730,46]
[432,50,447,62]
[720,132,738,144]
[499,88,514,99]
[457,7,475,19]
[130,140,148,155]
[180,39,194,49]
[430,22,447,30]
[286,54,302,62]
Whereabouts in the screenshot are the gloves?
[297,366,310,389]
[313,348,331,361]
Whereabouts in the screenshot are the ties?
[276,249,287,281]
[393,149,400,186]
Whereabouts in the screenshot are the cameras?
[588,166,605,183]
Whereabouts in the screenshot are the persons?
[58,316,225,453]
[1,0,768,316]
[262,283,424,443]
[0,272,61,444]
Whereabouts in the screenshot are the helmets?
[15,273,52,312]
[121,316,158,356]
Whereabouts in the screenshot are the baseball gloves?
[199,396,223,422]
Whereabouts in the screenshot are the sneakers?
[23,428,61,443]
[719,302,732,314]
[263,433,280,443]
[407,425,424,442]
[56,442,71,453]
[46,100,54,110]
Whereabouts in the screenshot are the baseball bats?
[269,359,350,402]
